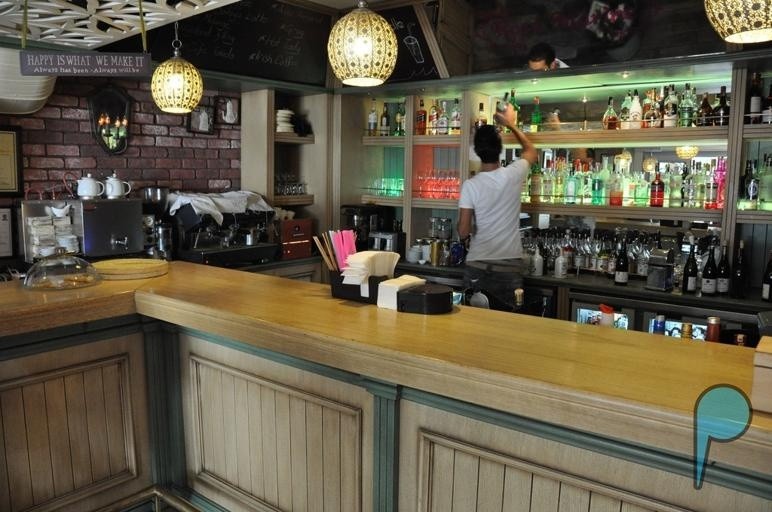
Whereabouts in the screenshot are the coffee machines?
[134,184,170,258]
[342,206,396,252]
[170,197,281,268]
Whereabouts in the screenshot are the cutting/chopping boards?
[91,259,169,280]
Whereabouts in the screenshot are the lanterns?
[704,0,772,43]
[151,57,202,113]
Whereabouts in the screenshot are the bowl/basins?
[51,206,70,216]
[45,206,55,216]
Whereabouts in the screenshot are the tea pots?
[199,109,212,131]
[106,174,132,200]
[221,101,237,124]
[154,222,173,261]
[76,173,105,199]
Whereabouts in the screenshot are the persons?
[455,105,536,292]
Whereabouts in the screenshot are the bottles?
[653,314,665,334]
[523,156,726,210]
[701,246,716,294]
[682,323,692,339]
[734,335,747,346]
[736,241,746,294]
[396,102,400,136]
[413,217,465,267]
[494,88,518,133]
[522,224,656,285]
[586,312,628,330]
[761,257,772,301]
[716,245,730,294]
[439,101,449,134]
[429,103,436,134]
[603,82,730,130]
[747,73,761,124]
[401,108,406,134]
[704,317,721,342]
[451,99,462,134]
[512,289,526,314]
[475,103,487,129]
[742,152,772,213]
[366,101,377,135]
[531,97,541,133]
[416,99,426,134]
[683,245,696,294]
[469,278,491,309]
[381,104,389,136]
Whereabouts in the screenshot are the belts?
[464,260,522,273]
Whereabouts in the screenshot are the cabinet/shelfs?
[239,91,334,250]
[331,54,772,348]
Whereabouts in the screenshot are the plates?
[28,216,56,258]
[54,216,79,253]
[275,109,294,133]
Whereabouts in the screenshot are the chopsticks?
[312,235,335,275]
[321,232,339,274]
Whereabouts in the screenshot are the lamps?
[701,1,772,47]
[98,112,130,152]
[150,21,203,114]
